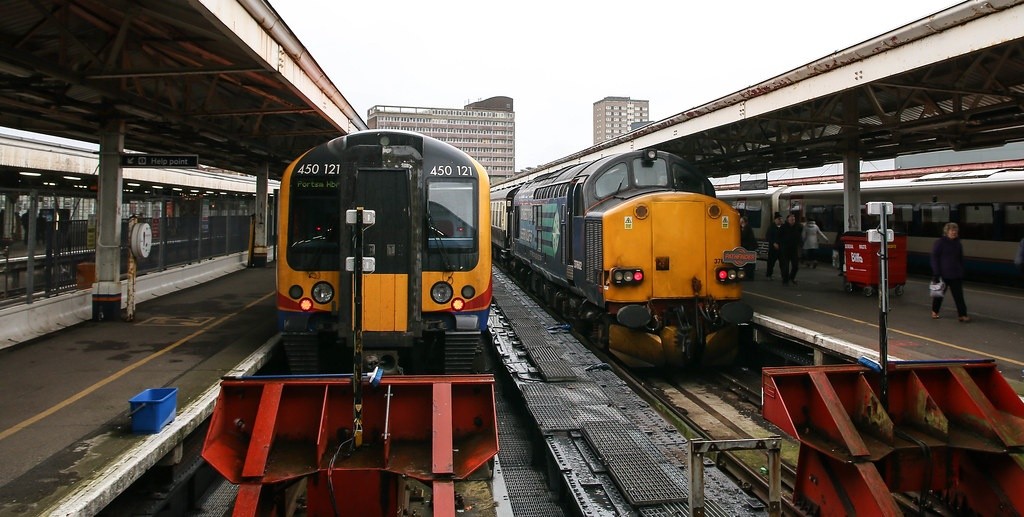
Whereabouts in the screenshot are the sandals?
[768,275,775,281]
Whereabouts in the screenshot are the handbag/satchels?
[929,277,946,298]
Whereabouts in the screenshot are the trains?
[709,168,1024,292]
[269,126,494,376]
[489,148,755,369]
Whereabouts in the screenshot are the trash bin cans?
[840,229,908,297]
[76,263,95,289]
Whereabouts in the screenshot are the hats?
[774,212,782,219]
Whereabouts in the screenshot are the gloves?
[934,273,945,282]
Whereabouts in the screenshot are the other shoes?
[782,281,788,286]
[931,311,939,319]
[807,263,811,266]
[813,262,817,269]
[959,316,971,322]
[792,278,797,283]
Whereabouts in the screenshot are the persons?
[928,223,971,321]
[766,212,803,287]
[737,214,757,283]
[801,217,828,270]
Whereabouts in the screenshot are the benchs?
[755,242,769,262]
